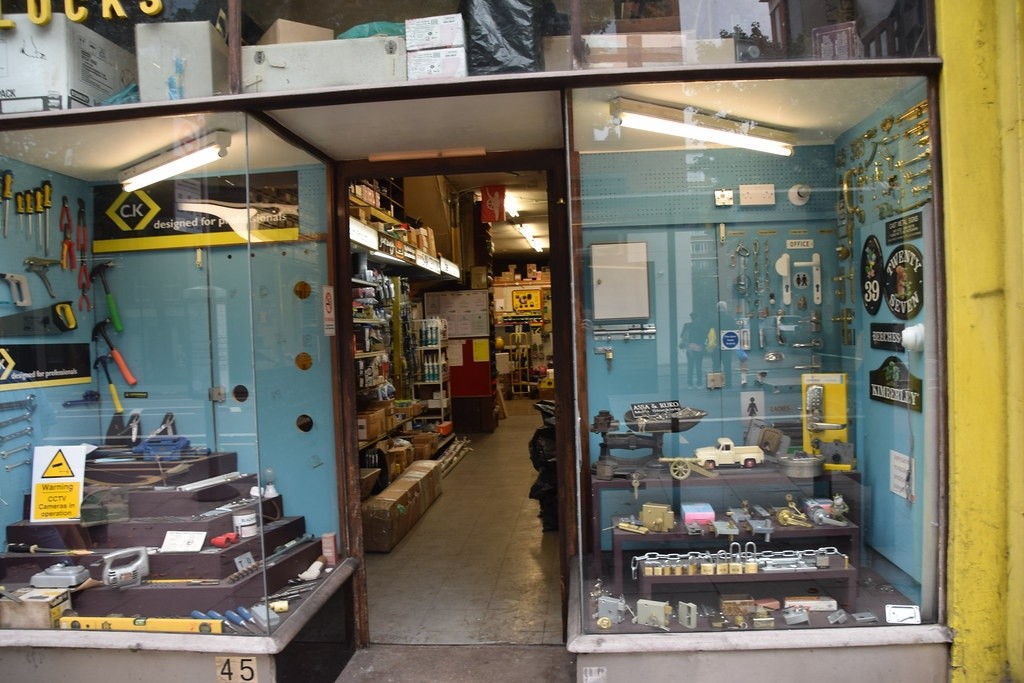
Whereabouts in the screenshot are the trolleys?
[658,456,718,481]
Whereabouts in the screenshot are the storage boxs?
[362,458,443,553]
[493,258,552,284]
[406,221,440,260]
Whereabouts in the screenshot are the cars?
[759,314,803,343]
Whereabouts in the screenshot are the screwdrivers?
[34,187,44,249]
[224,610,257,634]
[191,610,226,633]
[237,606,265,632]
[24,190,34,240]
[2,169,14,238]
[206,610,238,632]
[15,192,24,232]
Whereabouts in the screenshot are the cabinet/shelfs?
[590,449,866,615]
[405,317,453,436]
[349,241,399,452]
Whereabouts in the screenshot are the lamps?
[115,130,234,193]
[604,97,803,164]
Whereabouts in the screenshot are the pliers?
[78,245,90,290]
[22,257,59,298]
[61,223,77,270]
[116,414,141,442]
[79,283,91,312]
[145,414,176,441]
[60,195,73,233]
[77,197,88,251]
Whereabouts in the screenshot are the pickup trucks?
[694,438,766,471]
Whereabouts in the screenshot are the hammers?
[91,317,137,386]
[41,180,52,256]
[89,260,123,332]
[93,353,124,414]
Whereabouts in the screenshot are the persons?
[706,301,739,389]
[679,311,708,390]
